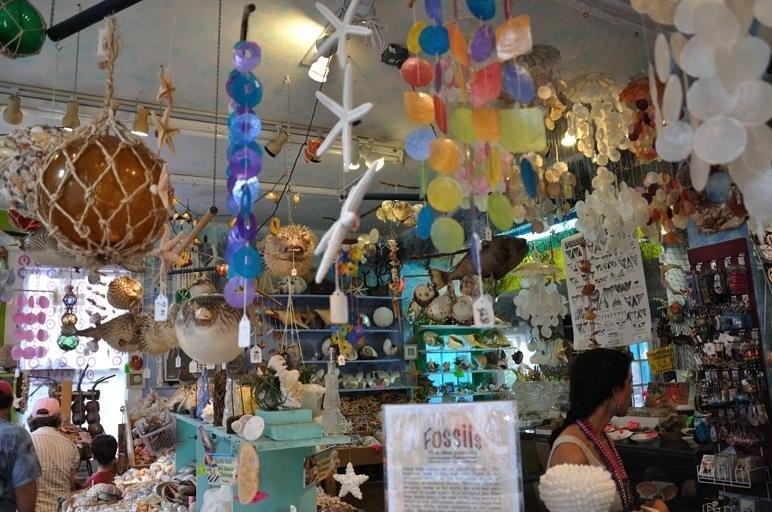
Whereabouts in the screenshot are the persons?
[26,396,80,512]
[83,434,118,491]
[544,347,639,510]
[1,379,44,512]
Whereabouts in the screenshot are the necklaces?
[575,415,637,511]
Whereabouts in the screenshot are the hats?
[32,398,61,417]
[0,380,12,397]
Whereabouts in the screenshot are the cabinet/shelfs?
[410,294,517,405]
[259,296,413,495]
[695,464,772,512]
[177,408,351,511]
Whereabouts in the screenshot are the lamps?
[63,98,82,132]
[307,55,332,84]
[264,132,287,158]
[359,145,384,171]
[1,97,24,125]
[132,110,152,138]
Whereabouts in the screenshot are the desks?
[532,435,712,512]
